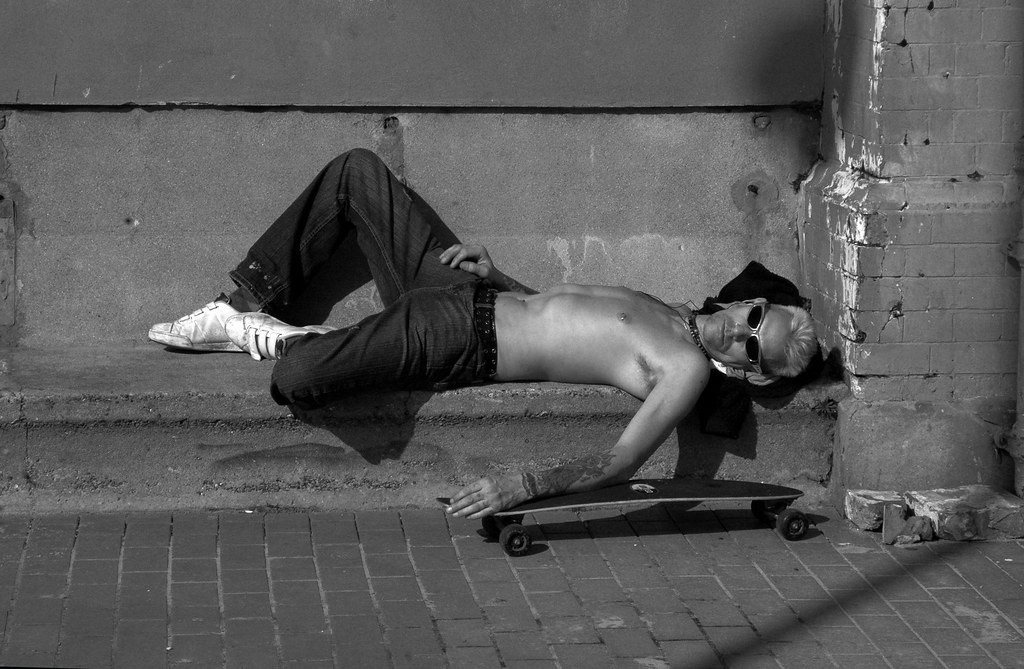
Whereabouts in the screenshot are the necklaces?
[688,311,711,361]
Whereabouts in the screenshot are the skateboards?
[434,477,810,557]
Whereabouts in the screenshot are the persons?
[147,147,821,521]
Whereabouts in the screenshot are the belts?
[474,288,497,383]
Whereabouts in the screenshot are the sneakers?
[224,312,338,361]
[147,292,262,353]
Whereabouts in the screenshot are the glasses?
[743,298,769,374]
